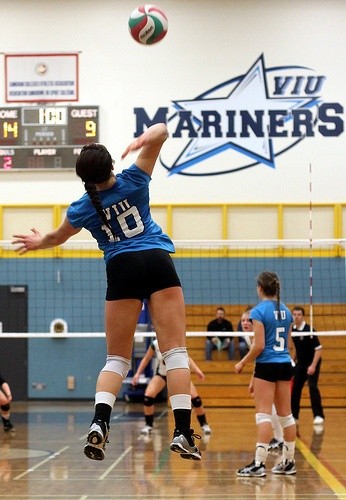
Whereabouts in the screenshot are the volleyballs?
[127,5,169,45]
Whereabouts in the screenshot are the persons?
[235,270,298,477]
[241,304,296,452]
[205,306,237,361]
[0,375,16,433]
[288,424,324,500]
[136,431,208,499]
[236,306,254,359]
[239,449,296,500]
[10,123,203,462]
[132,337,212,435]
[288,307,325,423]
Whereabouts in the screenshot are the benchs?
[183,302,346,409]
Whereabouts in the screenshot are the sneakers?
[202,424,210,433]
[269,437,284,452]
[169,429,203,461]
[312,415,324,424]
[84,420,109,461]
[272,460,297,474]
[235,460,267,476]
[141,425,152,434]
